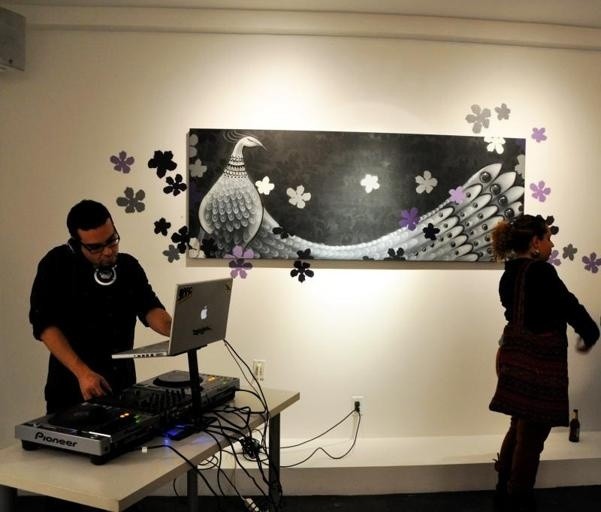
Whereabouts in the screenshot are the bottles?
[570,408,582,443]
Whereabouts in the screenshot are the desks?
[1,382,301,511]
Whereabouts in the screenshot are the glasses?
[79,225,120,254]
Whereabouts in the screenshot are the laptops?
[111,277,233,359]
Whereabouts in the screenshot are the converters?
[244,497,262,512]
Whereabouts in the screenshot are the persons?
[487,214,601,509]
[29,200,175,418]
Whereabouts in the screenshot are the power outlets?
[351,395,369,415]
[253,360,265,382]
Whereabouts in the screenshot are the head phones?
[67,237,119,288]
